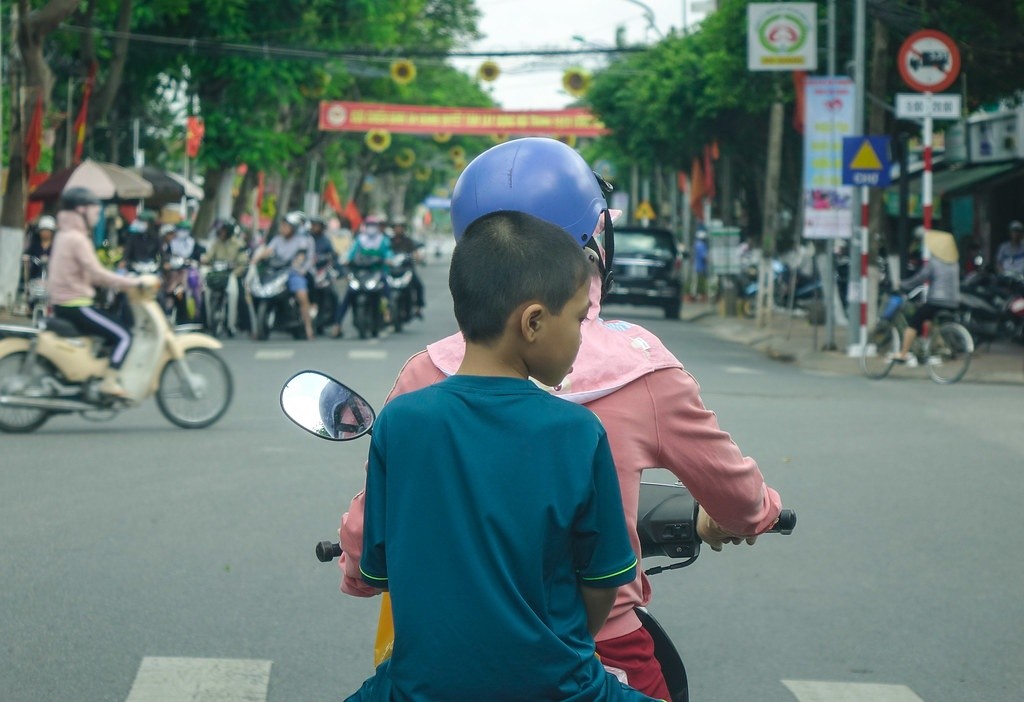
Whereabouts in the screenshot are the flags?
[187,115,204,158]
[689,151,715,220]
[324,182,361,229]
[76,66,97,159]
[26,94,48,219]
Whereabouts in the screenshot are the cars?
[607,227,685,320]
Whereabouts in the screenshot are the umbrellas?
[30,157,154,202]
[129,165,205,200]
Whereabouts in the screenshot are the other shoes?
[96,371,136,403]
[888,350,909,364]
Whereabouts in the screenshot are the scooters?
[0,275,234,433]
[13,239,426,338]
[743,236,1024,353]
[280,369,797,702]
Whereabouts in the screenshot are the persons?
[49,186,157,402]
[95,209,426,342]
[997,222,1024,279]
[342,210,665,702]
[337,137,783,702]
[894,230,960,363]
[731,238,752,317]
[23,216,56,308]
[320,384,371,438]
[692,230,708,301]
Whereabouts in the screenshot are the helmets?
[310,216,328,234]
[391,215,410,229]
[282,213,302,239]
[450,137,613,251]
[318,381,365,438]
[56,184,104,209]
[137,208,158,223]
[367,215,385,229]
[36,215,57,232]
[176,218,195,231]
[158,224,177,237]
[213,216,237,239]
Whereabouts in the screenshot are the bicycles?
[860,286,974,385]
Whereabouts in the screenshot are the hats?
[1008,219,1022,231]
